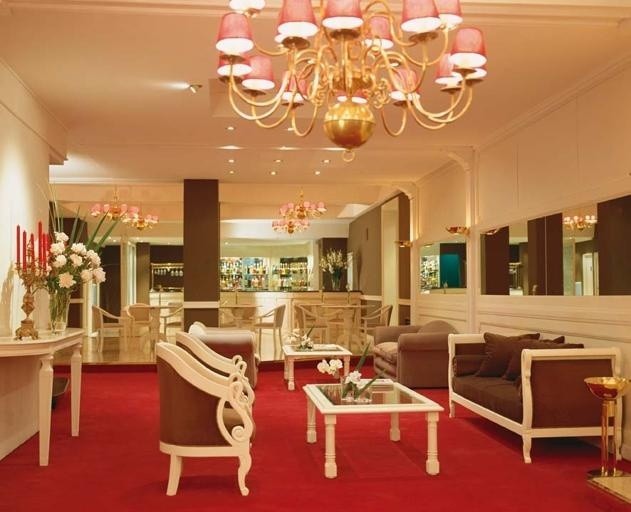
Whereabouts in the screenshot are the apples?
[13,221,48,275]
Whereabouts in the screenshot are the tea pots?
[479,194,631,298]
[418,234,468,297]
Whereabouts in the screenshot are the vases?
[36,180,129,324]
[319,250,354,289]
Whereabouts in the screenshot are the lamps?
[90,300,184,354]
[154,321,258,498]
[221,301,396,354]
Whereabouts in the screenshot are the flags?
[329,266,344,290]
[49,292,71,333]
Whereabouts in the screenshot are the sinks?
[375,322,622,466]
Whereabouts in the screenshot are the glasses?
[92,182,160,232]
[562,208,600,233]
[272,185,328,234]
[215,0,488,163]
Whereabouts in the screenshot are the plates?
[222,257,311,291]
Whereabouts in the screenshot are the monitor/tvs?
[362,379,395,393]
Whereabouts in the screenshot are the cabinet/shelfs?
[219,259,309,291]
